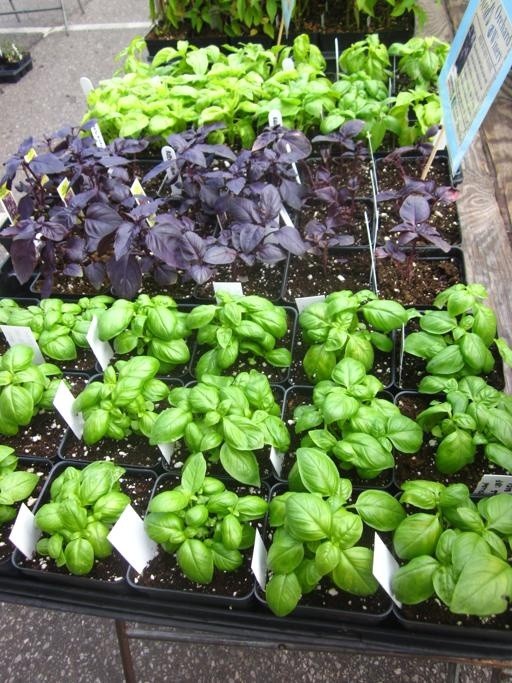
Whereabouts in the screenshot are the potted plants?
[2,1,511,651]
